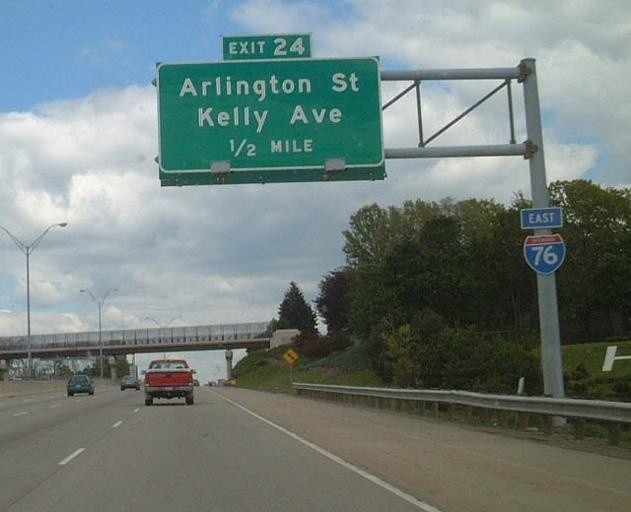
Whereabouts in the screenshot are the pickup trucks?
[142,359,196,409]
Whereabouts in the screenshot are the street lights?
[0,222,68,378]
[143,315,186,358]
[77,286,118,380]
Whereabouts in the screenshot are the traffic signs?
[222,34,313,60]
[156,55,384,174]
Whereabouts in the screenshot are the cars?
[119,375,141,392]
[65,375,96,397]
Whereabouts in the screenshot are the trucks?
[192,369,199,386]
